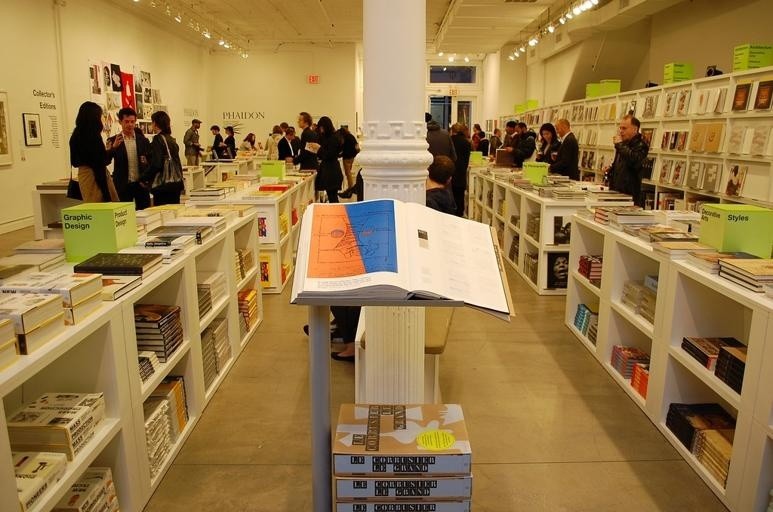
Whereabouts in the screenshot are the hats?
[192,119,202,123]
[224,127,233,130]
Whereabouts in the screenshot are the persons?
[421,101,653,222]
[68,100,366,213]
[302,315,346,344]
[329,306,362,364]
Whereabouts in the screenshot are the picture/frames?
[0,91,15,168]
[22,112,43,147]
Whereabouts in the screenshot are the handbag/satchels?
[151,159,181,190]
[66,180,84,200]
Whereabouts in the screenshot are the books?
[288,197,512,317]
[2,211,235,511]
[331,500,472,512]
[329,401,474,478]
[330,471,474,502]
[495,77,770,487]
[235,248,258,341]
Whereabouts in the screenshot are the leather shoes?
[331,352,355,361]
